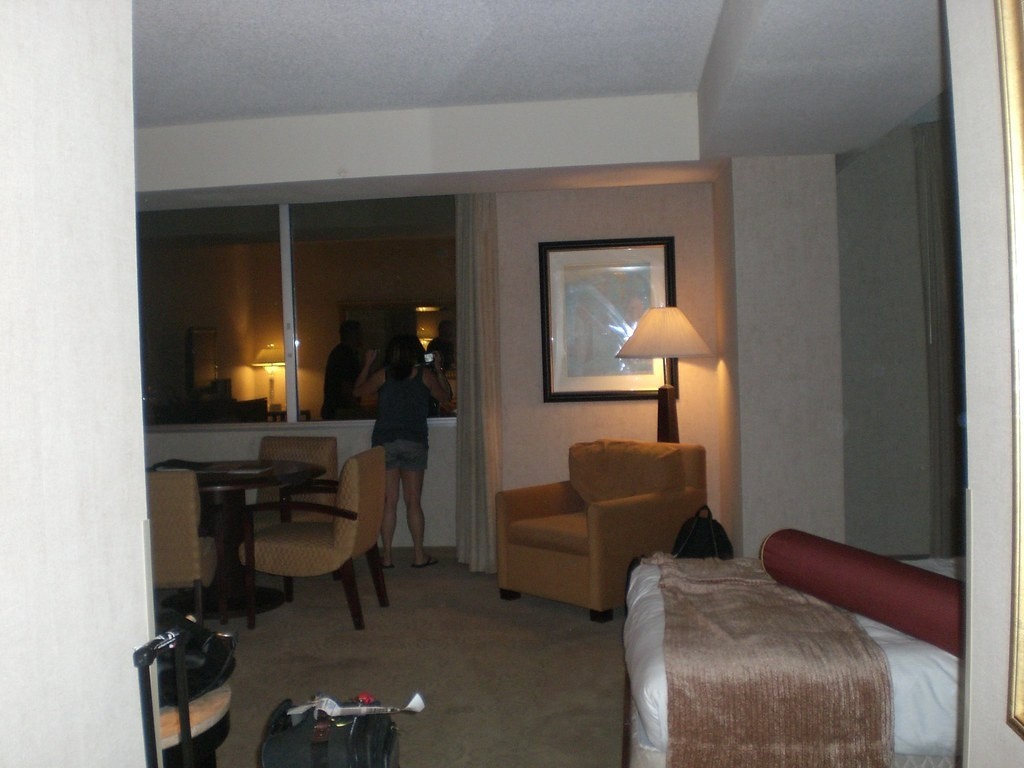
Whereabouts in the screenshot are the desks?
[146,458,326,622]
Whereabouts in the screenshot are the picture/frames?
[538,236,680,403]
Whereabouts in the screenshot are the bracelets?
[435,368,444,372]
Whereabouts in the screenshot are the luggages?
[260,699,399,768]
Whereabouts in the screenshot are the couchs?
[494,438,710,624]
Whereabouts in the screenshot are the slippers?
[383,564,394,568]
[412,557,439,567]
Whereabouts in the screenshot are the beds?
[621,551,966,768]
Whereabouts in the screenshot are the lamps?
[252,345,286,412]
[614,307,715,444]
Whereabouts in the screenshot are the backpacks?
[672,505,734,559]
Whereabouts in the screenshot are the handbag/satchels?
[155,607,236,710]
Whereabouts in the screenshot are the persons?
[320,319,373,421]
[425,320,458,373]
[352,332,453,570]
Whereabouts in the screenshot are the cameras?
[424,352,434,365]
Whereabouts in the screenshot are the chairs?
[145,436,390,632]
